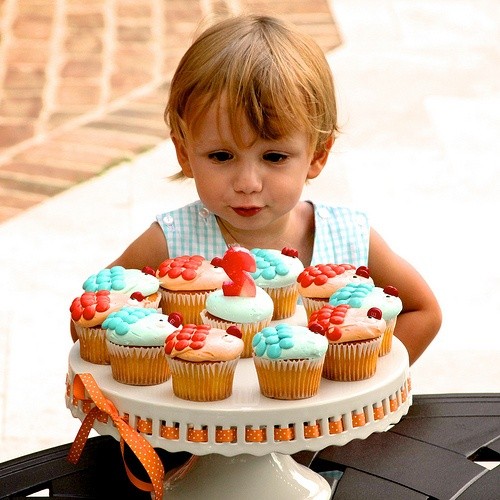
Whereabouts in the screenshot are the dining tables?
[0,393,500,500]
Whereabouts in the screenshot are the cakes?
[163,324,244,401]
[307,305,387,381]
[82,265,162,308]
[200,248,274,358]
[70,290,147,365]
[155,254,229,325]
[102,308,184,385]
[330,283,403,357]
[251,323,329,400]
[245,247,305,319]
[297,263,374,324]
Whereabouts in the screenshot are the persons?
[70,15,444,366]
